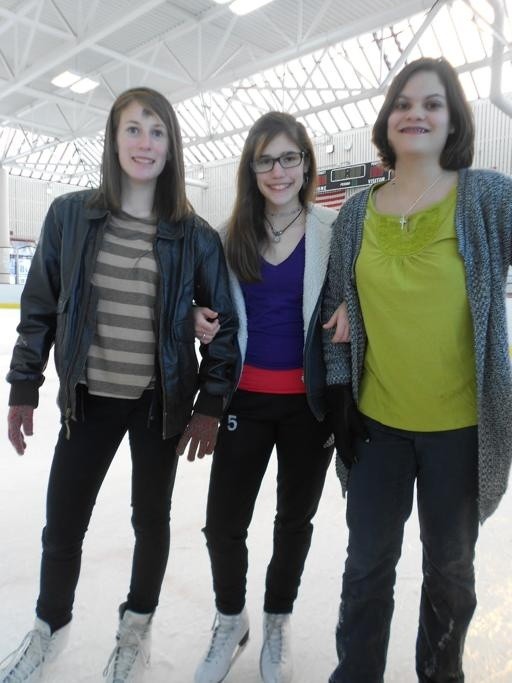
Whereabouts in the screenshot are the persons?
[317,58,511,683]
[192,111,351,683]
[1,86,241,683]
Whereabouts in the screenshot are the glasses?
[247,149,304,174]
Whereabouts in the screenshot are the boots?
[9,613,74,682]
[259,609,294,683]
[194,603,249,683]
[101,602,155,683]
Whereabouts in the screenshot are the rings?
[202,333,205,340]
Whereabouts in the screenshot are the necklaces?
[262,208,304,242]
[391,175,443,230]
